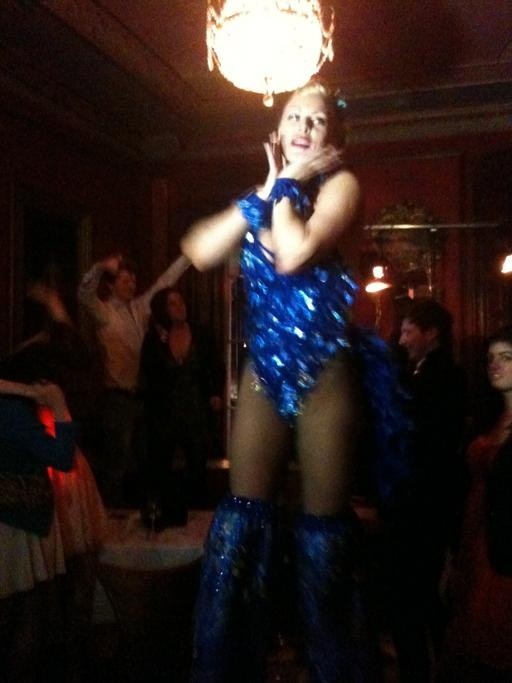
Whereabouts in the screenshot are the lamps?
[201,0,336,110]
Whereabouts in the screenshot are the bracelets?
[269,176,310,214]
[235,191,267,230]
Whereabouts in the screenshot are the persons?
[0,245,511,682]
[181,79,368,682]
